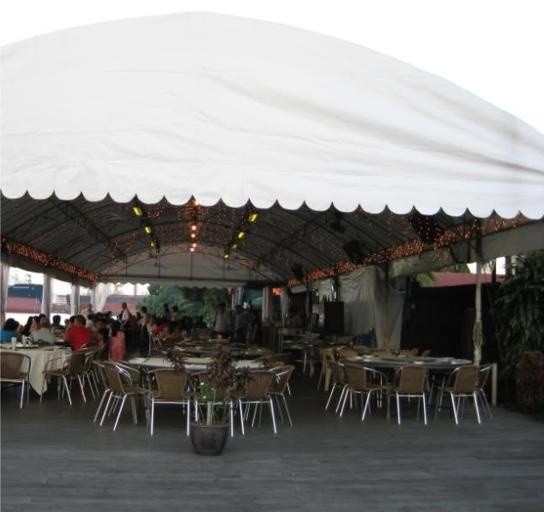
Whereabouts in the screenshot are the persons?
[136,300,206,356]
[122,302,132,331]
[30,317,56,345]
[51,315,64,331]
[211,301,258,344]
[107,321,127,361]
[64,315,91,350]
[38,314,47,328]
[81,302,94,319]
[96,319,109,344]
[0,319,20,344]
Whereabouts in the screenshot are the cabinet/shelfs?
[319,301,344,337]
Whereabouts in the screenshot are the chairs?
[147,369,191,436]
[235,370,278,435]
[317,348,335,392]
[91,359,142,425]
[434,365,482,426]
[100,360,151,432]
[1,352,31,408]
[387,364,427,426]
[339,363,388,422]
[255,365,296,428]
[69,350,100,400]
[39,352,87,405]
[324,360,367,413]
[115,360,142,384]
[191,369,236,438]
[461,363,493,421]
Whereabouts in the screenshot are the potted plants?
[159,348,255,456]
